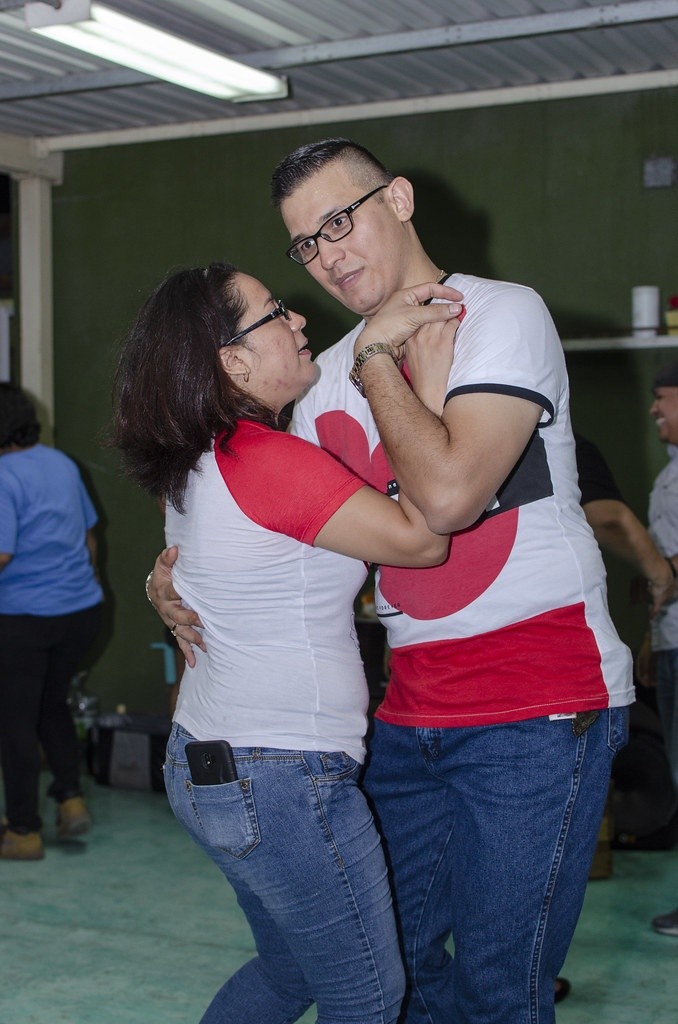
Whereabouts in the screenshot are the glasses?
[219,300,290,346]
[285,186,386,265]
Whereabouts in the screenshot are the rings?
[171,624,178,636]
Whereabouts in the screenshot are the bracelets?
[664,556,677,578]
[145,570,153,602]
[646,568,673,592]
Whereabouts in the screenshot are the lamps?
[29,0,289,103]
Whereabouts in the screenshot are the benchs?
[97,710,173,793]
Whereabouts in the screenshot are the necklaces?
[422,270,446,305]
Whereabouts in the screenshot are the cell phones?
[184,739,238,786]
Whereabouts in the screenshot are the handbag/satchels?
[81,712,168,793]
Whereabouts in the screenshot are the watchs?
[348,343,400,399]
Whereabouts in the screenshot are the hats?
[654,362,678,387]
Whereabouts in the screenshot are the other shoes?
[553,977,572,1003]
[55,798,91,835]
[653,907,678,936]
[0,826,44,861]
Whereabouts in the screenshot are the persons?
[578,357,678,938]
[0,380,107,864]
[144,137,635,1024]
[114,261,459,1024]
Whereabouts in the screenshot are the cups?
[631,285,660,329]
